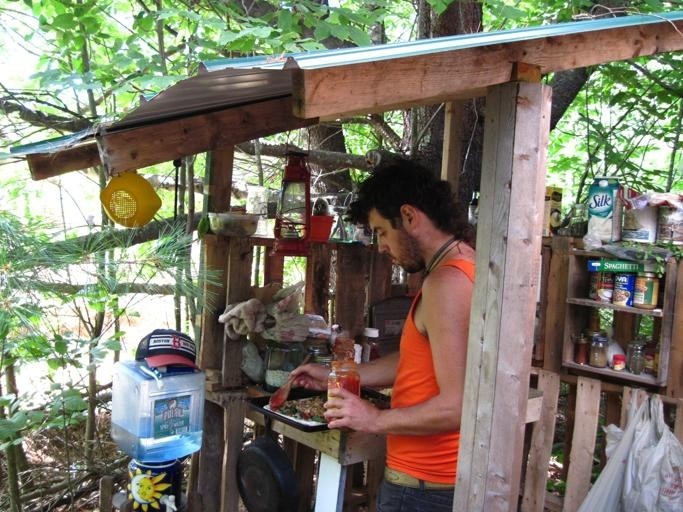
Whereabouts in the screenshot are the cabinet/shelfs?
[562,246,678,388]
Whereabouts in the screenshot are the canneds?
[578,271,659,310]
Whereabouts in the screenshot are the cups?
[245,185,270,237]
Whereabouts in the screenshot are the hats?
[135,328,201,372]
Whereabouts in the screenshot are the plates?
[262,395,328,427]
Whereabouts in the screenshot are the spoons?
[267,352,313,408]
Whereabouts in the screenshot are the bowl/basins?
[206,211,259,238]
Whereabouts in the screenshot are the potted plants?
[309,197,336,241]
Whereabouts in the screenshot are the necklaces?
[417,233,464,279]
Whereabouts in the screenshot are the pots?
[234,413,302,512]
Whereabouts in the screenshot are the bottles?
[334,336,355,360]
[628,345,646,376]
[588,335,608,368]
[573,337,589,365]
[361,327,381,364]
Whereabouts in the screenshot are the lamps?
[268,126,316,260]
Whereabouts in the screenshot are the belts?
[381,464,455,489]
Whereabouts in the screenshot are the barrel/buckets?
[111,361,205,462]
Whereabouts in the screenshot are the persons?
[286,154,476,512]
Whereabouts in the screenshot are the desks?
[205,384,542,512]
